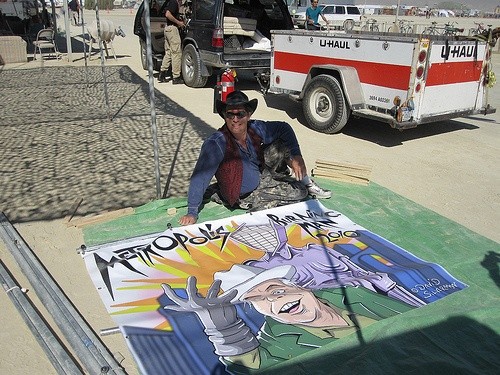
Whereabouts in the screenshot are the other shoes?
[157,72,167,82]
[172,76,186,84]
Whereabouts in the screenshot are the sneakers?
[305,179,332,199]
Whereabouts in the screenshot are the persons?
[158,0,185,83]
[177,90,332,227]
[68,0,82,25]
[306,0,329,31]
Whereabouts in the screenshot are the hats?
[216,91,258,117]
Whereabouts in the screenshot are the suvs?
[132,0,296,88]
[293,4,362,31]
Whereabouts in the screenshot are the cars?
[113,0,137,9]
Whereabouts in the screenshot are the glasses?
[226,111,248,119]
[313,1,318,3]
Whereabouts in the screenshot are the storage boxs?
[222,16,256,36]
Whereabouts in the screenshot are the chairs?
[32,29,59,65]
[88,19,117,61]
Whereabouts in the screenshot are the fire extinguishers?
[213,62,235,114]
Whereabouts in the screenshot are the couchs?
[0,36,27,63]
